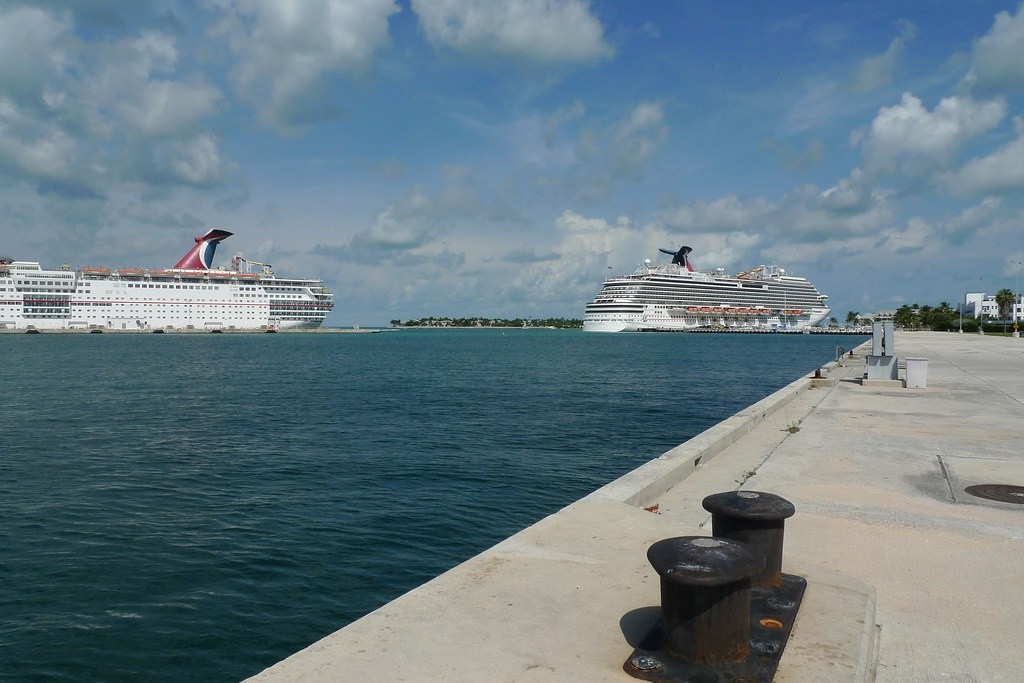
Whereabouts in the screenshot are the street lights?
[1011,260,1021,331]
[980,277,983,330]
[960,287,970,329]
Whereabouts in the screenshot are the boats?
[207,272,235,279]
[80,266,110,275]
[148,269,178,278]
[686,307,802,316]
[180,271,205,280]
[237,272,260,281]
[115,269,145,276]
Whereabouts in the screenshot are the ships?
[1,228,334,328]
[582,245,832,332]
